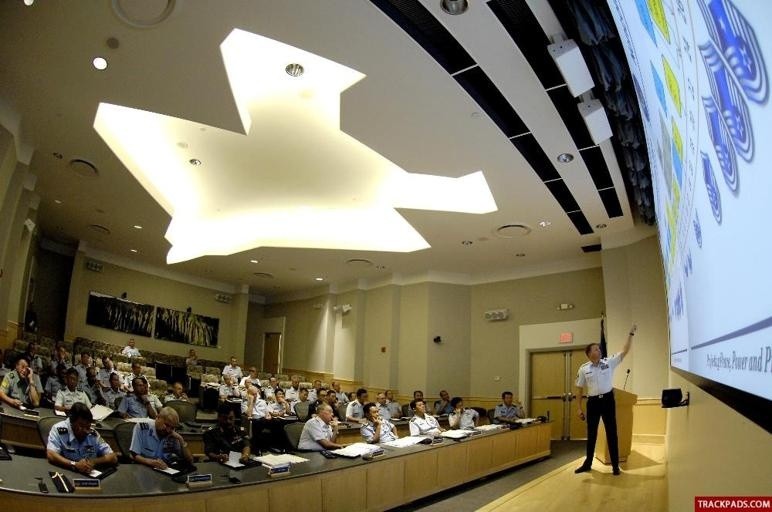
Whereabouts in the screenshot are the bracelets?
[519,407,523,409]
[629,332,634,336]
[180,441,187,449]
[70,461,76,471]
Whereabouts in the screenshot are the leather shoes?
[612,466,620,475]
[574,463,592,474]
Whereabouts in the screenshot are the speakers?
[215,293,233,304]
[87,260,105,273]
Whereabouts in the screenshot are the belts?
[588,388,613,400]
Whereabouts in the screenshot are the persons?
[128,406,193,470]
[0,339,200,421]
[202,348,479,465]
[25,302,39,334]
[492,391,525,424]
[574,320,640,475]
[46,402,118,476]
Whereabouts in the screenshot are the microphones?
[623,368,631,391]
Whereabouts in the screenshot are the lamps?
[216,294,232,303]
[87,261,102,271]
